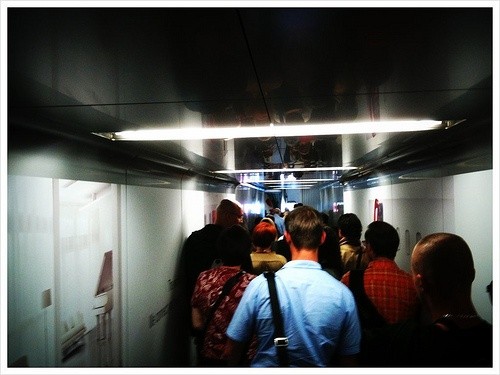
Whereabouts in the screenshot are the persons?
[333,212,371,275]
[176,194,493,369]
[246,218,286,274]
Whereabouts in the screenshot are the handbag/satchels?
[348,267,384,352]
[191,327,206,345]
